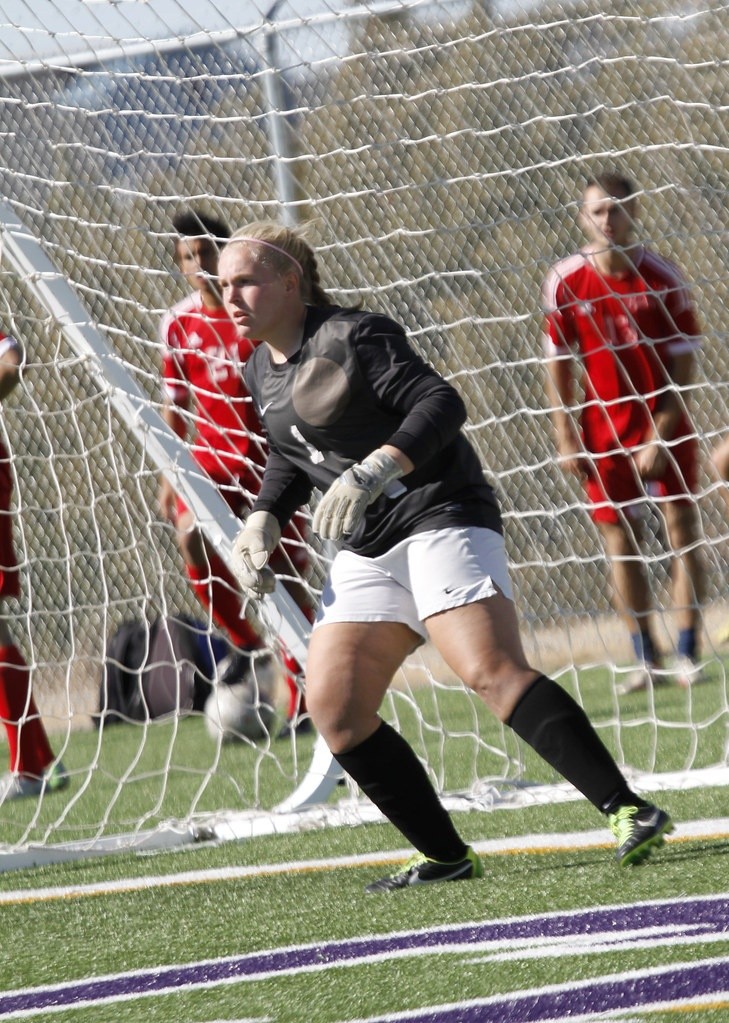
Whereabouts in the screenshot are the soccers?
[204,682,273,742]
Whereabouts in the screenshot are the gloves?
[311,449,405,542]
[230,511,281,600]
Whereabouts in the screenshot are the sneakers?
[274,714,311,739]
[671,658,708,688]
[613,659,665,696]
[0,757,70,801]
[606,804,676,867]
[363,844,485,896]
[219,637,273,684]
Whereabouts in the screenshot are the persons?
[91,613,231,731]
[218,224,675,890]
[0,329,71,802]
[542,174,705,695]
[159,210,315,740]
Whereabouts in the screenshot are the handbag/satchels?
[93,613,229,726]
